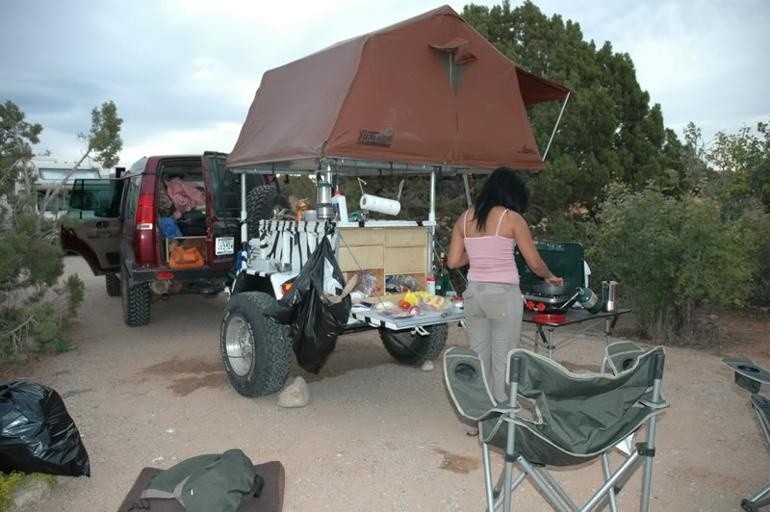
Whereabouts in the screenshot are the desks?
[512,306,629,361]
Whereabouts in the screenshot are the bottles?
[436,264,449,296]
[600,280,618,312]
[426,275,436,295]
[575,286,602,313]
[332,191,349,222]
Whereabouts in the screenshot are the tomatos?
[399,301,410,311]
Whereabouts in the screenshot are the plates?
[530,314,565,323]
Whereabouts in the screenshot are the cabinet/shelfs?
[332,223,425,303]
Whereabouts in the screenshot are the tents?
[226,5,577,173]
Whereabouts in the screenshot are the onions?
[411,305,422,317]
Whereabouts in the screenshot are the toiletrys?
[330,185,349,223]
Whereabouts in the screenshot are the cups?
[304,210,316,220]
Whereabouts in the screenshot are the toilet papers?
[359,194,401,216]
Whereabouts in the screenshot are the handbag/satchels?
[169,247,204,270]
[140,449,265,512]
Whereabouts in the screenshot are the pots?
[533,283,568,296]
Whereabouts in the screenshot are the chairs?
[718,347,770,512]
[438,334,663,511]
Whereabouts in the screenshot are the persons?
[446,167,564,404]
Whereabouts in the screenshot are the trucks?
[0,155,104,258]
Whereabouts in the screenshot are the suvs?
[59,151,292,327]
